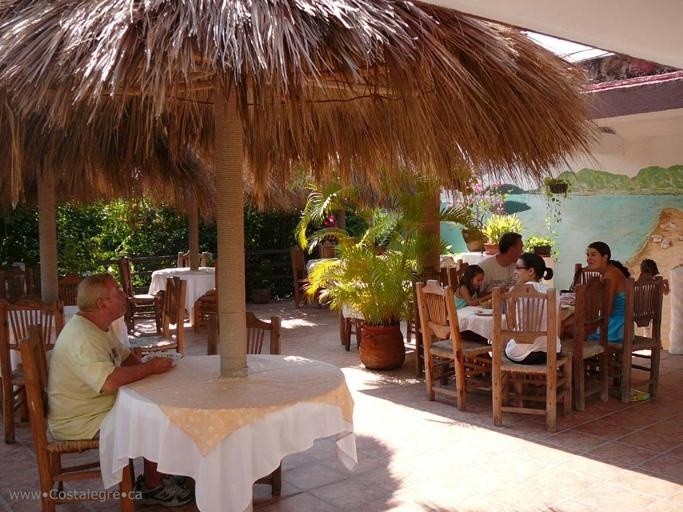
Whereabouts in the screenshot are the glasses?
[514,264,524,271]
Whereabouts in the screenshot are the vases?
[459,227,487,252]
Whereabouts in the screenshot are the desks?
[116,352,359,512]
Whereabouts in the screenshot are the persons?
[47,272,196,508]
[563,242,636,362]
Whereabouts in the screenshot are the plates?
[141,352,184,368]
[474,309,493,316]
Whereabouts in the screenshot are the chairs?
[205,310,283,500]
[287,238,385,352]
[20,324,136,512]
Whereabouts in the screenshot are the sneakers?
[132,475,195,506]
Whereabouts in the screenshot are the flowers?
[446,163,503,228]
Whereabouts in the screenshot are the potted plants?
[524,236,559,258]
[479,214,526,256]
[542,175,573,235]
[293,169,471,372]
